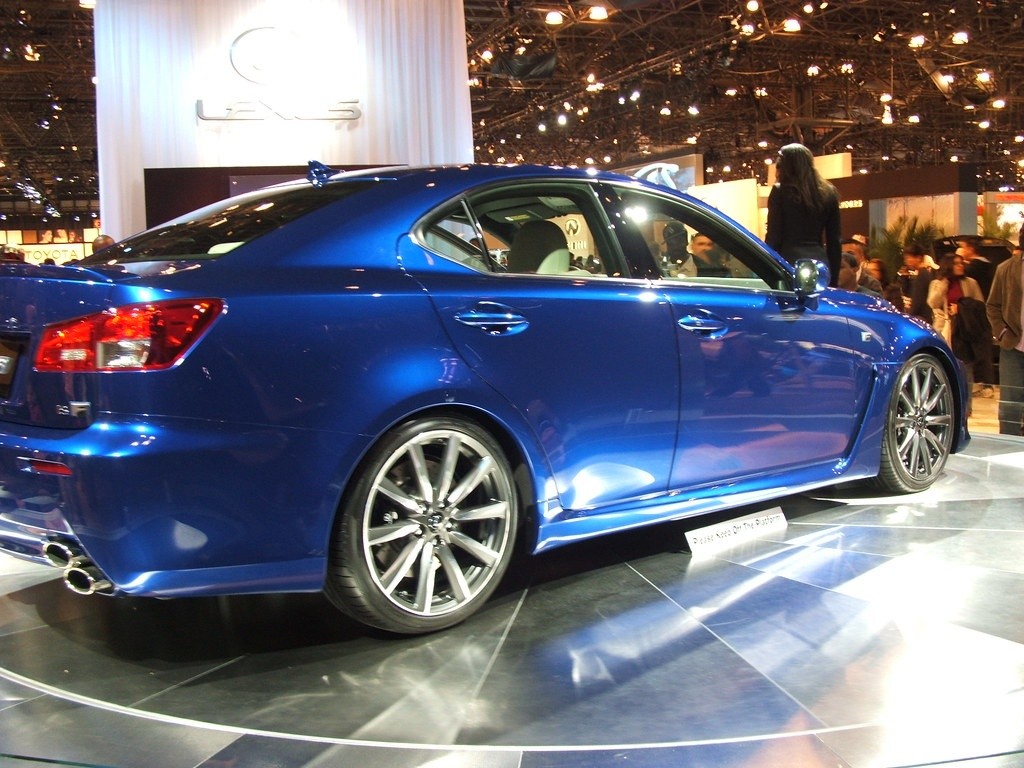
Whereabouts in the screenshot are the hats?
[660,222,686,244]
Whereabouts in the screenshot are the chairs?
[508,219,570,275]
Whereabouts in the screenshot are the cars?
[929,233,1021,284]
[486,248,512,269]
[1,159,974,636]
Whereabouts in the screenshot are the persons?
[985,222,1024,435]
[468,221,732,280]
[762,144,841,286]
[44,234,116,265]
[838,237,996,385]
